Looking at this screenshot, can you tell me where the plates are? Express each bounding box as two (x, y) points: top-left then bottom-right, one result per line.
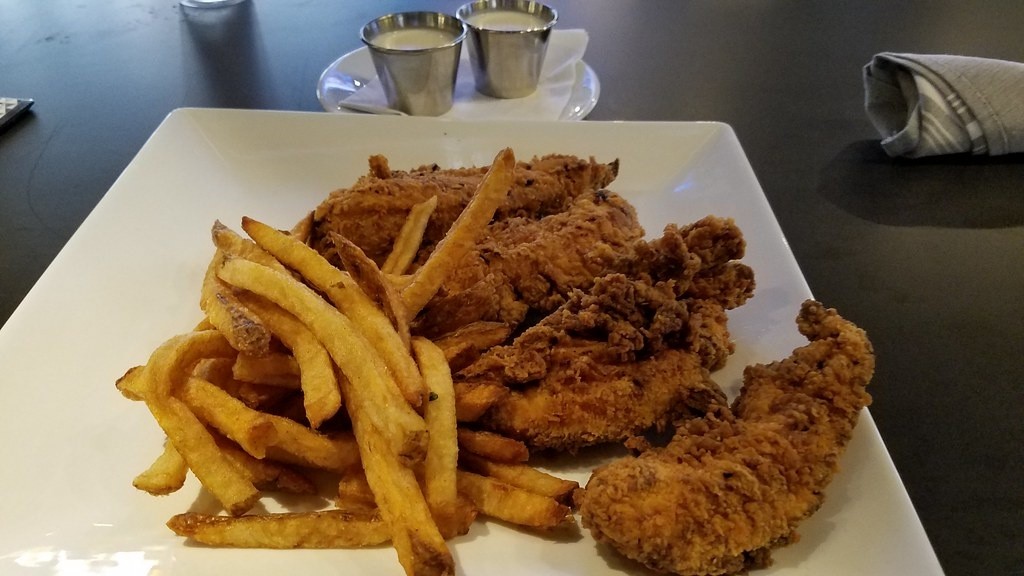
(316, 45), (601, 121)
(0, 108), (946, 576)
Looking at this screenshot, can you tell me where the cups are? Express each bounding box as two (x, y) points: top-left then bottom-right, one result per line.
(456, 0), (559, 99)
(360, 11), (468, 117)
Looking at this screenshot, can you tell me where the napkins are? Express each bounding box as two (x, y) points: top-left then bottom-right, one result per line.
(861, 52), (1024, 158)
(338, 27), (590, 121)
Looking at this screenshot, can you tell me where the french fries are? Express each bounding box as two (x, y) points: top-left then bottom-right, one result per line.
(115, 147), (582, 576)
